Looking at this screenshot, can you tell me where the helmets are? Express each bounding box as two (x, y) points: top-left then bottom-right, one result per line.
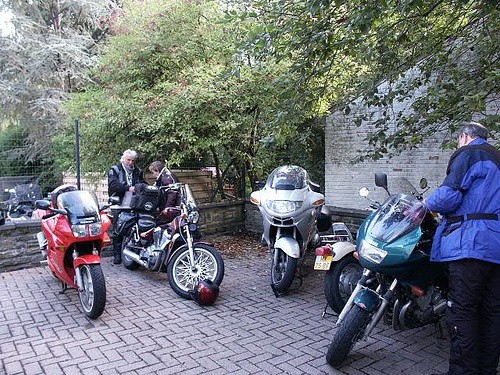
(194, 278), (219, 306)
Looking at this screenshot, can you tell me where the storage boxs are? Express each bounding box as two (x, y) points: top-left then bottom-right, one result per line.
(120, 190), (160, 215)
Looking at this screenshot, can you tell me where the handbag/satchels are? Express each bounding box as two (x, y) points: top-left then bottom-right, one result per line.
(115, 212), (137, 236)
(130, 183), (159, 213)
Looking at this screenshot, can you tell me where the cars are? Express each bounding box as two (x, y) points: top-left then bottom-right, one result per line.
(201, 167), (222, 179)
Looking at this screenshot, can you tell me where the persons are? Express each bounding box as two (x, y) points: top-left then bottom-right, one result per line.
(149, 160), (182, 219)
(419, 123), (500, 375)
(107, 149), (143, 265)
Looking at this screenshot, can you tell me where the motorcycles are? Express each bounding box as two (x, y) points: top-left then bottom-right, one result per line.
(325, 172), (452, 368)
(110, 182), (225, 301)
(313, 177), (431, 318)
(249, 165), (332, 294)
(0, 184), (54, 225)
(34, 184), (121, 320)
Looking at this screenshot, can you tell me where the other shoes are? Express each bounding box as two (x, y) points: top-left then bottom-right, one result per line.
(114, 255), (122, 264)
